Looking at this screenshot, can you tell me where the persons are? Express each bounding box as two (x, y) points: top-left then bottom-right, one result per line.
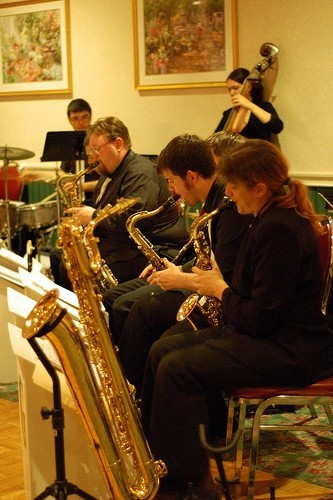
(81, 133), (114, 209)
(116, 133), (254, 396)
(58, 99), (101, 201)
(101, 129), (250, 344)
(214, 68), (284, 142)
(49, 117), (194, 300)
(139, 139), (333, 500)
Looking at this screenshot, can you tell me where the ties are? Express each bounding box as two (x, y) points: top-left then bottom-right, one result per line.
(94, 177), (111, 206)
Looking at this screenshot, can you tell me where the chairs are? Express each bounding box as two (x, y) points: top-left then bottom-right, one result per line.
(225, 215), (333, 499)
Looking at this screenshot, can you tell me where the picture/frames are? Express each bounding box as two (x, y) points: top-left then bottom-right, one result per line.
(131, 0), (241, 91)
(0, 0), (73, 96)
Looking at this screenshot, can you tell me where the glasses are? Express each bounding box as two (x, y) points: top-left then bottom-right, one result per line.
(89, 137), (115, 158)
(69, 116), (90, 122)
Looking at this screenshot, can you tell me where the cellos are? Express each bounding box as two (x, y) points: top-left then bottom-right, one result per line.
(222, 41), (279, 136)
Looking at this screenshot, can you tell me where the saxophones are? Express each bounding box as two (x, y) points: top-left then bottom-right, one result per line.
(19, 192), (169, 499)
(58, 162), (99, 291)
(81, 197), (141, 295)
(175, 194), (236, 335)
(126, 191), (181, 291)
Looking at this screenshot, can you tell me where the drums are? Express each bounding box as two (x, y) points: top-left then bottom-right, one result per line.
(0, 198), (62, 246)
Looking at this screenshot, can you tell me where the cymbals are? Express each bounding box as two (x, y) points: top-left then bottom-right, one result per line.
(0, 145), (36, 163)
